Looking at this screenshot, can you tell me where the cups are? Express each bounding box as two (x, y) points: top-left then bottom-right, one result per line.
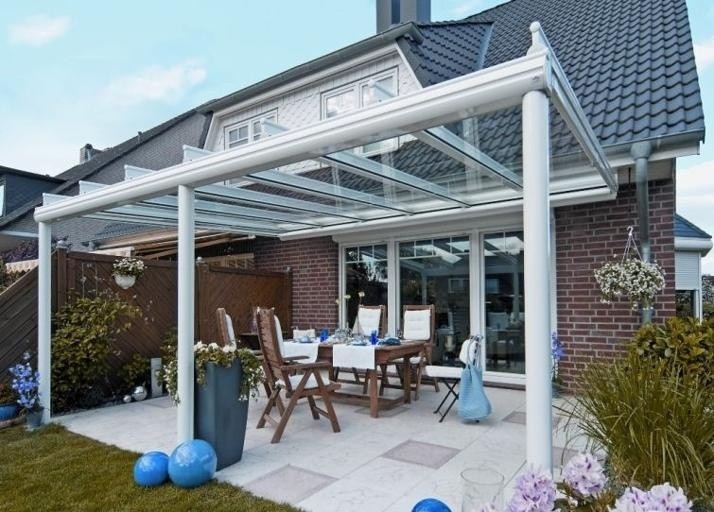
(457, 467), (505, 511)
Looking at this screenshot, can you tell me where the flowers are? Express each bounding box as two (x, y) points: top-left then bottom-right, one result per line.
(152, 341), (267, 403)
(8, 352), (41, 418)
(110, 258), (148, 279)
(594, 255), (667, 312)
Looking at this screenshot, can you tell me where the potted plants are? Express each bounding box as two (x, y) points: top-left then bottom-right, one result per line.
(0, 376), (19, 421)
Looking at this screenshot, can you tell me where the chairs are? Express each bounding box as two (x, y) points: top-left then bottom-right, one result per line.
(425, 337), (479, 422)
(379, 304), (439, 401)
(215, 307), (276, 407)
(256, 306), (341, 443)
(328, 304), (389, 394)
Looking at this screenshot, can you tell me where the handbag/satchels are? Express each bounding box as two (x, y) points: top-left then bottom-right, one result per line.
(458, 367), (490, 420)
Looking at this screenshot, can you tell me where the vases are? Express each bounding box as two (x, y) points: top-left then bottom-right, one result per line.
(114, 274), (136, 290)
(26, 405), (44, 426)
(193, 356), (250, 472)
